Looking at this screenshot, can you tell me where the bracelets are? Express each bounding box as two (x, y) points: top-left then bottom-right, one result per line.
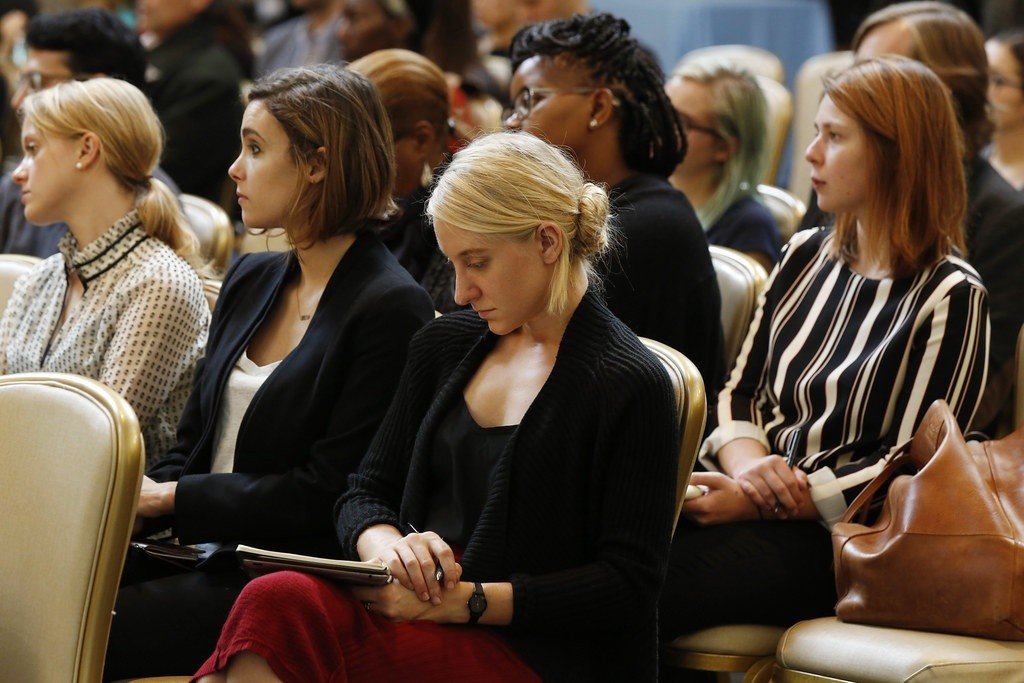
(758, 504), (764, 520)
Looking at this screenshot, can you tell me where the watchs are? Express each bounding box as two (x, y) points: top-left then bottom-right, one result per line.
(467, 582), (487, 625)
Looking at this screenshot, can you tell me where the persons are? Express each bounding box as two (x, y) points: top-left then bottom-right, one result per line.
(0, 0), (1024, 683)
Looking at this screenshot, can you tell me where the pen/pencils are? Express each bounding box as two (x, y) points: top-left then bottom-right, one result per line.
(774, 427), (803, 513)
(406, 520), (444, 581)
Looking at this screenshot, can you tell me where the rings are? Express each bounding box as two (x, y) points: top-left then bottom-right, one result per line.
(364, 602), (371, 611)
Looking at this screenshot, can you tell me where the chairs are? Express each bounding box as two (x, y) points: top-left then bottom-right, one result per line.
(0, 40), (1024, 683)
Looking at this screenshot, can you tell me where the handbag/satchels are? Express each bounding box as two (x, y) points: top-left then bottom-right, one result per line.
(831, 398), (1024, 641)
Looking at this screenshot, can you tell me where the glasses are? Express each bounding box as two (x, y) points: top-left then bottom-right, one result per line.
(677, 107), (727, 141)
(13, 66), (96, 92)
(495, 84), (629, 124)
(988, 69), (1023, 90)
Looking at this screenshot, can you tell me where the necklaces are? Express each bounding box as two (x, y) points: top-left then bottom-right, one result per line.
(297, 283), (321, 321)
